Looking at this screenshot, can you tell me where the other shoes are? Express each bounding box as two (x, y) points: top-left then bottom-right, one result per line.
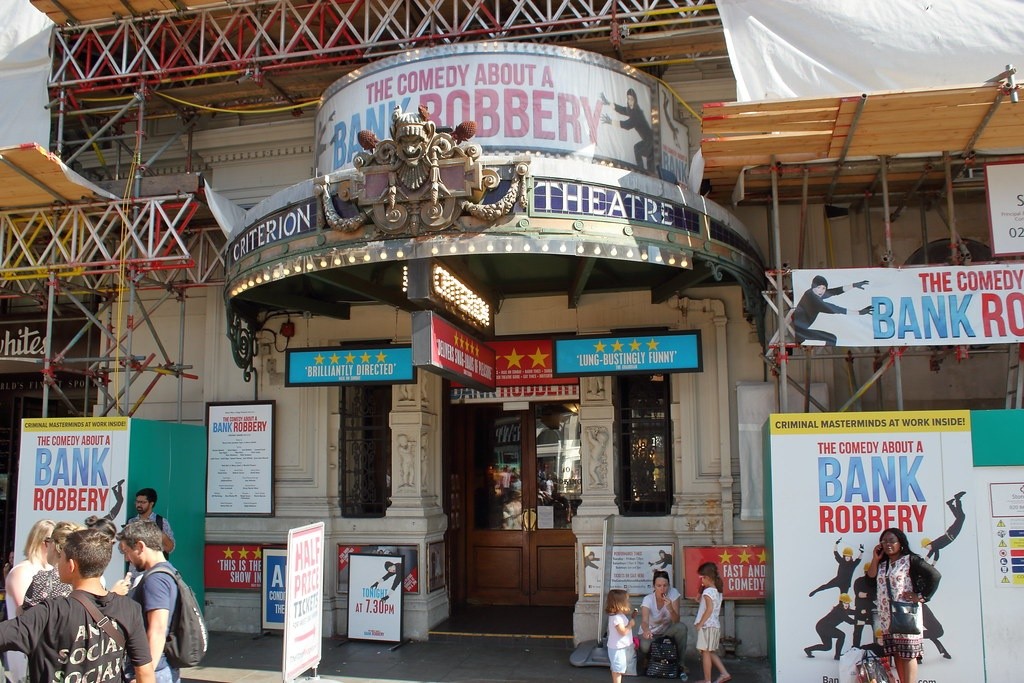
(713, 674), (732, 683)
(804, 648), (815, 657)
(809, 591), (816, 596)
(943, 651), (951, 658)
(955, 492), (966, 499)
(947, 498), (955, 505)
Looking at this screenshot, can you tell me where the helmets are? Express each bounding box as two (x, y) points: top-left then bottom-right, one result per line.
(921, 538), (932, 546)
(864, 562), (872, 571)
(844, 548), (853, 556)
(839, 592), (852, 603)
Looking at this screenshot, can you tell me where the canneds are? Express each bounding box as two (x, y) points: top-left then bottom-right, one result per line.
(680, 672), (687, 682)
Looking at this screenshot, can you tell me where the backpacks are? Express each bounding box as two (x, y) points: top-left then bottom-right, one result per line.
(141, 566), (209, 667)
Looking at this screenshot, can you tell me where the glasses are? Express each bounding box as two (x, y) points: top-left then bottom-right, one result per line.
(699, 575), (706, 579)
(45, 538), (51, 547)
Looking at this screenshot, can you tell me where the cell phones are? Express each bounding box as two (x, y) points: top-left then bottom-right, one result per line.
(124, 572), (132, 582)
(878, 548), (883, 553)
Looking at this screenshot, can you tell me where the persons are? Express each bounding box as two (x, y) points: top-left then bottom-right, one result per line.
(102, 479), (126, 520)
(693, 560), (732, 682)
(604, 588), (634, 683)
(488, 462), (559, 496)
(125, 488), (177, 589)
(790, 273), (869, 347)
(636, 570), (690, 673)
(802, 490), (968, 664)
(0, 514), (183, 682)
(660, 87), (680, 148)
(599, 88), (656, 175)
(862, 527), (941, 683)
(368, 559), (402, 602)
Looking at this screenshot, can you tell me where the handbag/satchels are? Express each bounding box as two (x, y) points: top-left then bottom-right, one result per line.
(889, 599), (921, 635)
(838, 647), (898, 683)
(646, 636), (679, 678)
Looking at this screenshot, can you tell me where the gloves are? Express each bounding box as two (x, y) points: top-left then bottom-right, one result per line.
(601, 113), (612, 124)
(370, 582), (379, 589)
(648, 562), (654, 566)
(328, 110), (336, 122)
(858, 305), (875, 316)
(865, 619), (875, 626)
(653, 568), (657, 572)
(853, 280), (869, 290)
(859, 544), (864, 553)
(599, 91), (610, 106)
(835, 537), (842, 545)
(381, 595), (389, 602)
(330, 135), (337, 146)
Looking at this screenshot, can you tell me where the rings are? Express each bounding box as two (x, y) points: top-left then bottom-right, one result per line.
(647, 634), (650, 637)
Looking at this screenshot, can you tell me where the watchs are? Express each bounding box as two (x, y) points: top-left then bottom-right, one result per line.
(917, 593), (927, 604)
